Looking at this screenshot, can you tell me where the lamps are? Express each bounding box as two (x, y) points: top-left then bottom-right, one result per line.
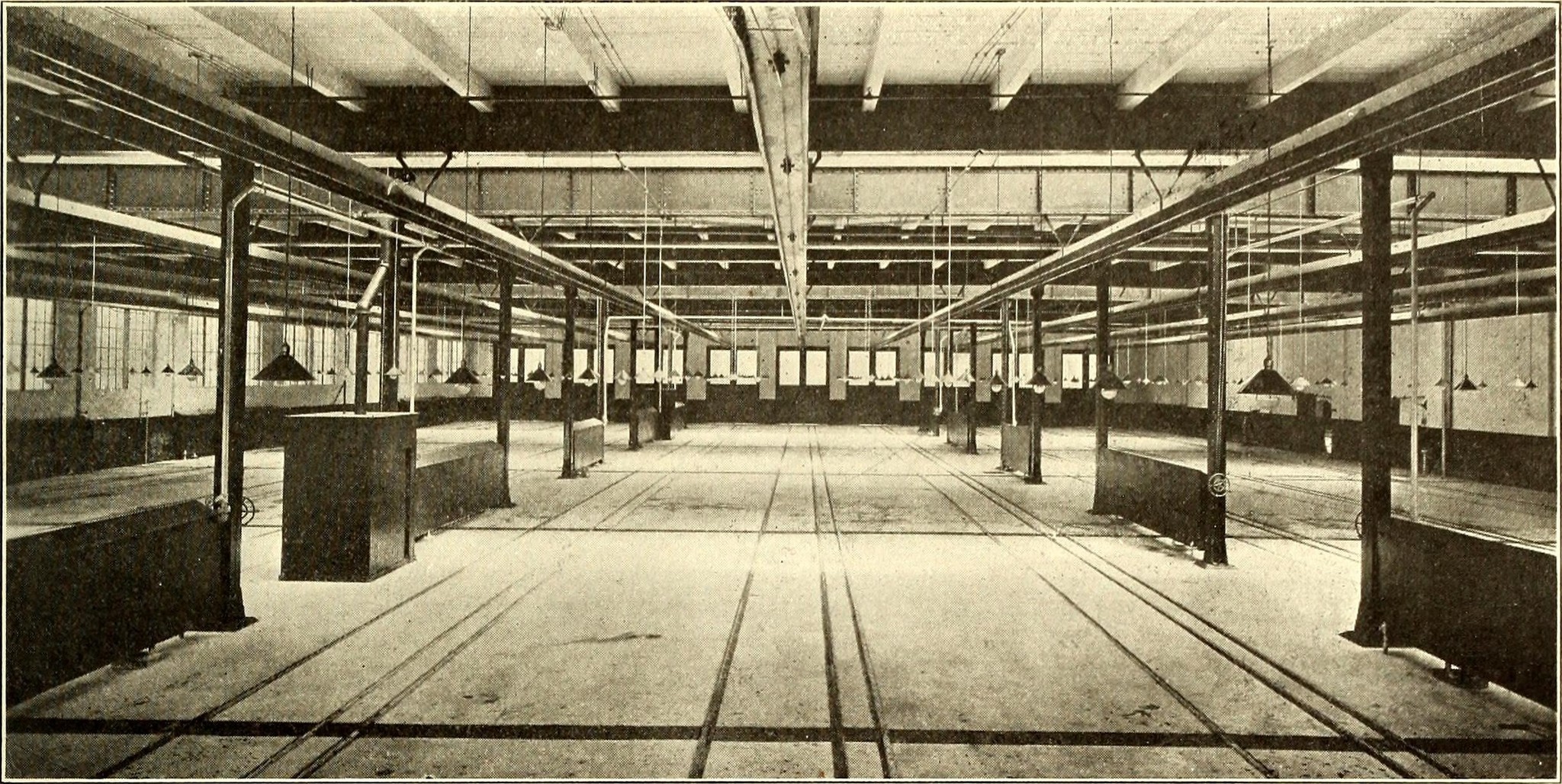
(7, 7), (1556, 398)
